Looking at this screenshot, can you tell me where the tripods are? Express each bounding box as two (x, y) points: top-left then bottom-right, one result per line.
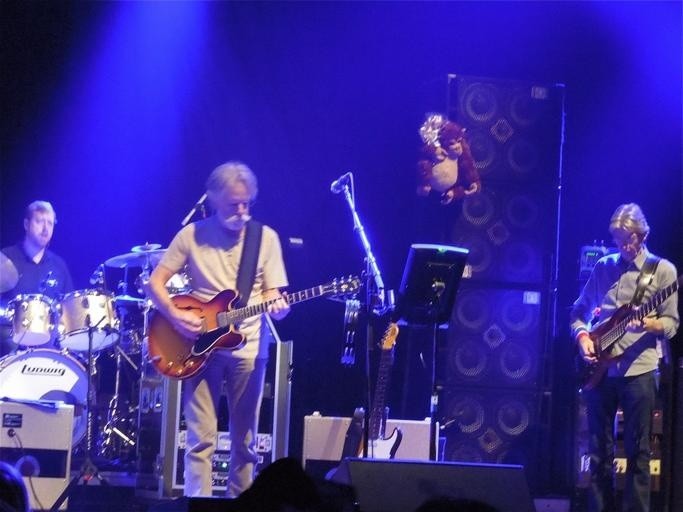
(73, 255), (149, 458)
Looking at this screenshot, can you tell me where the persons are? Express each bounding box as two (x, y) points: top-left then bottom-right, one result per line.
(0, 201), (75, 358)
(569, 202), (680, 511)
(144, 162), (290, 500)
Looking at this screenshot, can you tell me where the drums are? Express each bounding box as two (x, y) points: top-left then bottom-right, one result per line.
(7, 294), (57, 346)
(55, 287), (121, 353)
(0, 348), (96, 451)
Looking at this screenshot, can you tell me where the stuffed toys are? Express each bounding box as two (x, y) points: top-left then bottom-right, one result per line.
(417, 114), (481, 205)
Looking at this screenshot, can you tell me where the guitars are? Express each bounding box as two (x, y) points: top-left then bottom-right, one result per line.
(574, 273), (683, 394)
(325, 324), (402, 486)
(149, 274), (364, 380)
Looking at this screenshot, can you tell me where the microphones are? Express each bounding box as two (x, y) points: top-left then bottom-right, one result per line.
(181, 190), (208, 226)
(39, 272), (51, 293)
(331, 173), (350, 194)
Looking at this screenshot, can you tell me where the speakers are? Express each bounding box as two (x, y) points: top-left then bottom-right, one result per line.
(436, 72), (566, 500)
(328, 457), (536, 512)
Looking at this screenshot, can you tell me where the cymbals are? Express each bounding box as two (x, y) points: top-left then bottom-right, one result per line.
(131, 243), (161, 252)
(104, 249), (167, 268)
